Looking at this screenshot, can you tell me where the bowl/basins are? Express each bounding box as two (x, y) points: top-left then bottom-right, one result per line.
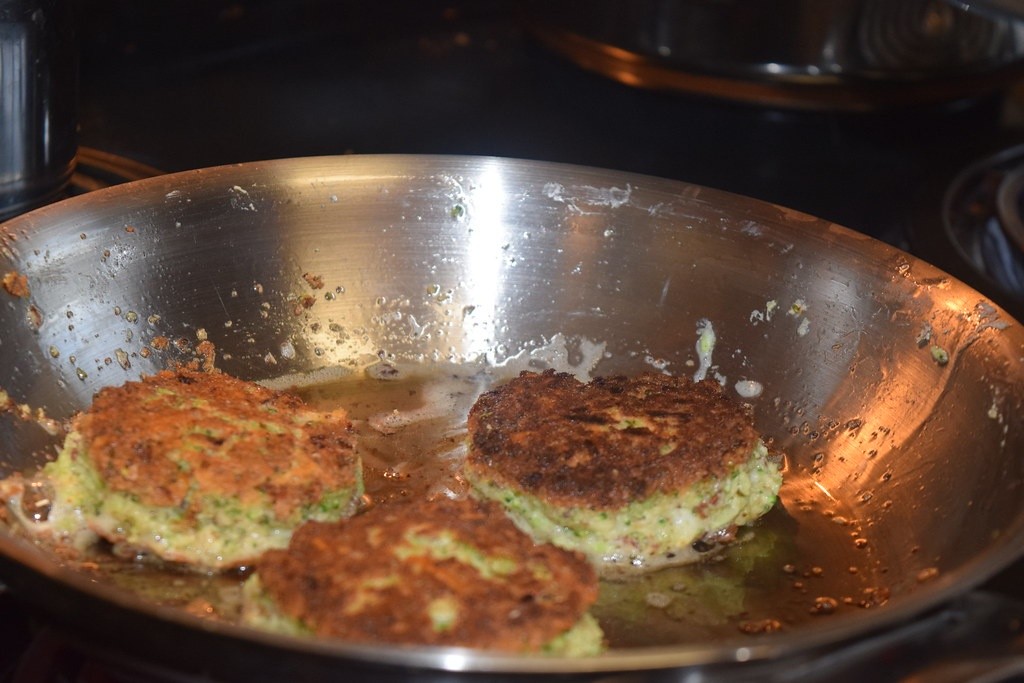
(0, 154), (1024, 683)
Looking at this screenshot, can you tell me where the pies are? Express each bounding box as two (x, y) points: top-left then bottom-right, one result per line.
(46, 367), (364, 575)
(463, 370), (784, 569)
(243, 494), (609, 657)
